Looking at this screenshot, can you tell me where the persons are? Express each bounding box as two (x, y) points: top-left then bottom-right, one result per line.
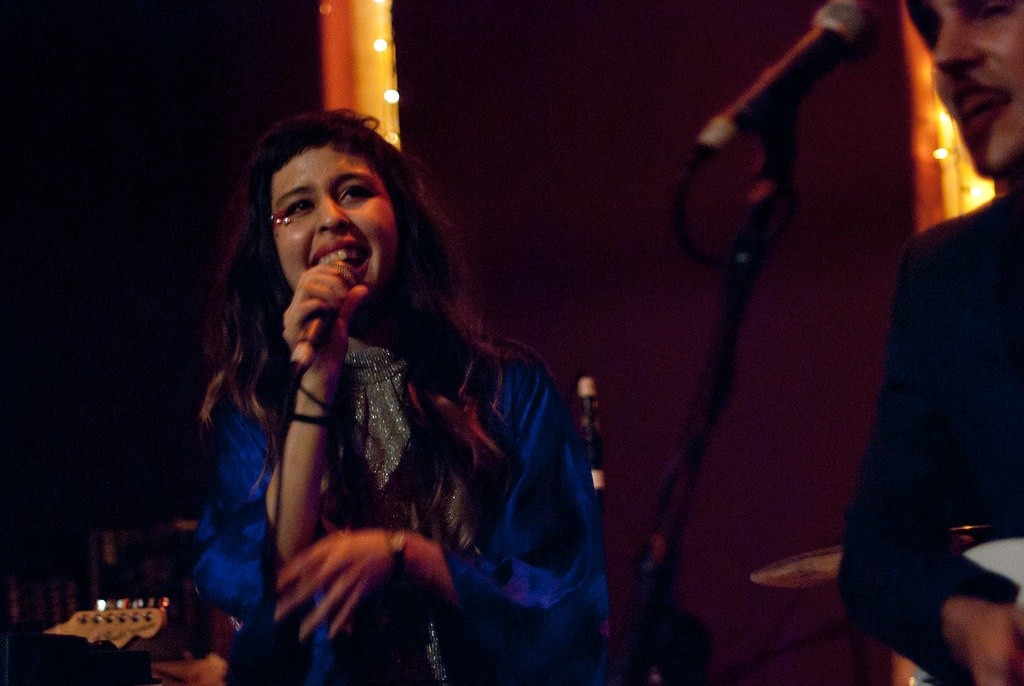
(836, 0), (1024, 686)
(193, 108), (610, 686)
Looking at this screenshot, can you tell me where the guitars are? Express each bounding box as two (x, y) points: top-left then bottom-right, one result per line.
(42, 595), (171, 649)
(892, 537), (1024, 686)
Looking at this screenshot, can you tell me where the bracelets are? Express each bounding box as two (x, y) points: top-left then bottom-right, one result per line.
(389, 527), (406, 581)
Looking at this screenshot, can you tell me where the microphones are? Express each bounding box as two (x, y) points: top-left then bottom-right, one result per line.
(287, 260), (358, 379)
(579, 373), (607, 513)
(691, 0), (881, 164)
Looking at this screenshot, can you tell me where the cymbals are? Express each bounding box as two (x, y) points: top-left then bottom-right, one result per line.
(749, 523), (994, 588)
